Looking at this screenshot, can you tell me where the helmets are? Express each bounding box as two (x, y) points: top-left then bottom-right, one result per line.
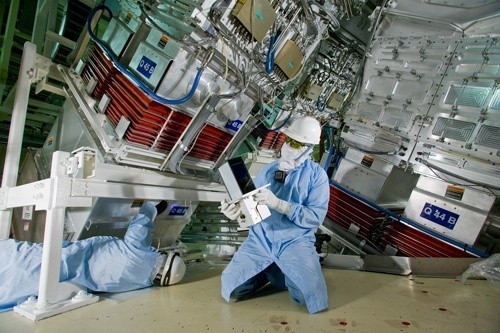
(160, 250), (186, 285)
(284, 116), (321, 144)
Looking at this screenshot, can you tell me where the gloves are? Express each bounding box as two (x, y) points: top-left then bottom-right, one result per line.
(221, 198), (242, 220)
(253, 188), (288, 215)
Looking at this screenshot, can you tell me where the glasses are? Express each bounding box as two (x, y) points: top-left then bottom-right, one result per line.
(286, 136), (311, 149)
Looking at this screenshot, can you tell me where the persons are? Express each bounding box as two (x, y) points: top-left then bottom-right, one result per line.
(0, 199), (186, 312)
(221, 116), (330, 313)
